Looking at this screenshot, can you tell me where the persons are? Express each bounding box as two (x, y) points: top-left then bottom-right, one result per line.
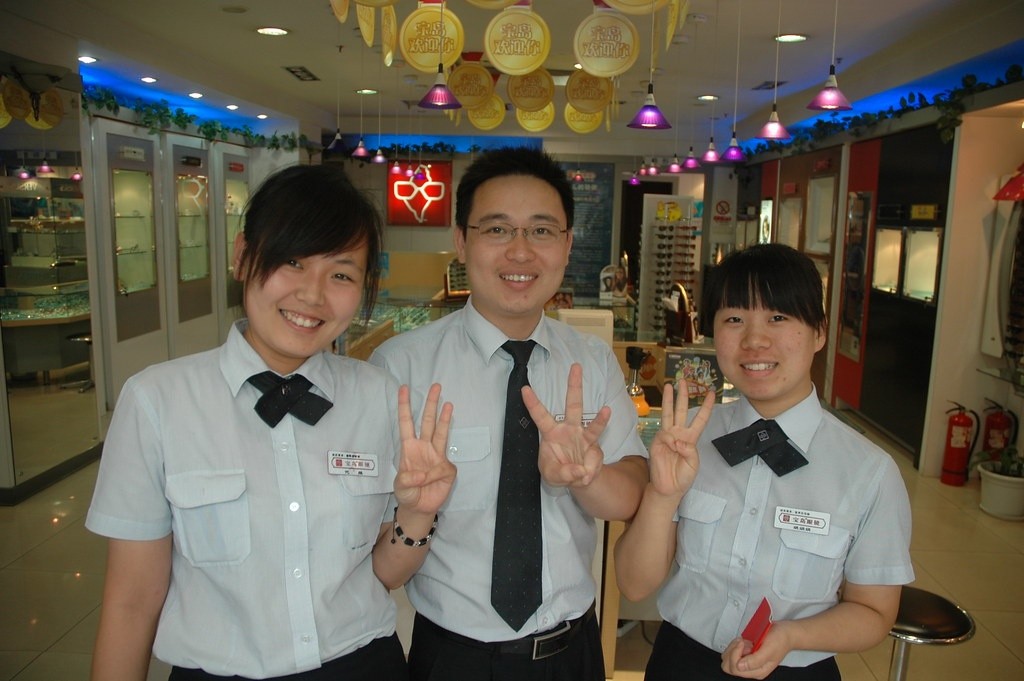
(367, 143), (653, 681)
(613, 243), (916, 681)
(84, 164), (457, 681)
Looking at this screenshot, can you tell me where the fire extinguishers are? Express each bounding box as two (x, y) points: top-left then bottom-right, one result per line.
(941, 400), (981, 487)
(978, 396), (1019, 482)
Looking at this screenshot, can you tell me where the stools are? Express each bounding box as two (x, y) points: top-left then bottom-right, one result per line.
(890, 585), (976, 681)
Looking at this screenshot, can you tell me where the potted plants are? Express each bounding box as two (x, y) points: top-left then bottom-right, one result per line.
(968, 443), (1024, 522)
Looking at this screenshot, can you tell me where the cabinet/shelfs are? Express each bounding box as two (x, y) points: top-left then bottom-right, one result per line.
(0, 176), (92, 376)
(601, 343), (726, 680)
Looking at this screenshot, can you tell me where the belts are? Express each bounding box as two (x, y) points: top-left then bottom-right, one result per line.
(415, 598), (596, 660)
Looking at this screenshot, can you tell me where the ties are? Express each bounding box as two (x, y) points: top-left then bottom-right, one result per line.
(491, 339), (543, 634)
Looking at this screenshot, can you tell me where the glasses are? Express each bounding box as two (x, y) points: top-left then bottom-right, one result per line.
(0, 209), (246, 320)
(464, 222), (568, 245)
(360, 257), (470, 326)
(633, 218), (697, 330)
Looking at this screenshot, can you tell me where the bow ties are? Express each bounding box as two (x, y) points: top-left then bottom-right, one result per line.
(710, 419), (809, 477)
(247, 371), (334, 429)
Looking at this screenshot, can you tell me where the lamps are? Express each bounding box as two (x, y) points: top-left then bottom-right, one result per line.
(328, 0), (462, 180)
(628, 0), (851, 184)
(16, 118), (83, 182)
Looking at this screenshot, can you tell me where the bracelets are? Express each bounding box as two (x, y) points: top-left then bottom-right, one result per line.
(391, 506), (438, 547)
